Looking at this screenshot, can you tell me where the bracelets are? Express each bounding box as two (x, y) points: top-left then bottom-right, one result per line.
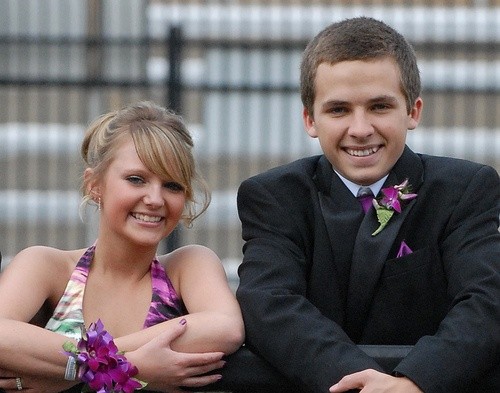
(63, 333), (83, 382)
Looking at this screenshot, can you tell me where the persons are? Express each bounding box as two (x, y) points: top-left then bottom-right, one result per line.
(0, 101), (245, 393)
(237, 16), (499, 393)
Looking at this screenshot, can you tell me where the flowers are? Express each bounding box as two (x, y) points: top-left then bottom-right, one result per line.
(60, 316), (148, 393)
(371, 178), (418, 236)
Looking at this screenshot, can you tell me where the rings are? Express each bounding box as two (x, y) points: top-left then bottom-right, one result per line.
(16, 377), (22, 390)
